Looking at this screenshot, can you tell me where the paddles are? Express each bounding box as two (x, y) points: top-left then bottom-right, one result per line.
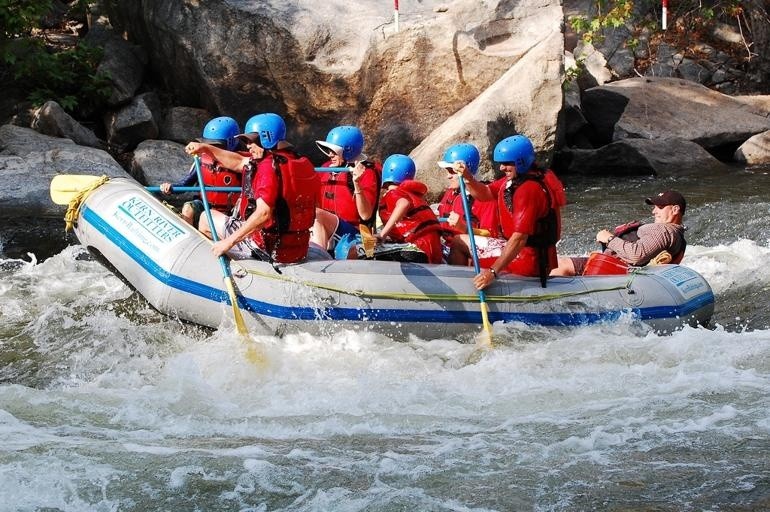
(189, 145), (265, 368)
(456, 163), (499, 351)
(50, 174), (242, 205)
(359, 224), (392, 259)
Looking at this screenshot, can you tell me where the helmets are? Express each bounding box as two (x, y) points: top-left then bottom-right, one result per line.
(435, 142), (481, 180)
(200, 114), (242, 153)
(492, 134), (537, 175)
(316, 124), (366, 161)
(380, 152), (417, 190)
(233, 110), (288, 150)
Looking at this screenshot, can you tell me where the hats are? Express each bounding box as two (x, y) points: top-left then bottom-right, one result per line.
(643, 189), (687, 210)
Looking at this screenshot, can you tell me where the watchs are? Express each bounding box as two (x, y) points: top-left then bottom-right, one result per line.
(607, 235), (615, 246)
(489, 267), (497, 278)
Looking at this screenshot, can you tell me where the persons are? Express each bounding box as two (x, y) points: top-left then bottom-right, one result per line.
(186, 113), (321, 264)
(454, 134), (565, 291)
(436, 143), (497, 249)
(160, 116), (246, 229)
(550, 191), (688, 276)
(312, 125), (383, 260)
(375, 153), (443, 267)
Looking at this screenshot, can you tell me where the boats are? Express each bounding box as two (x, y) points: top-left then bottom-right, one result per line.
(70, 177), (715, 336)
